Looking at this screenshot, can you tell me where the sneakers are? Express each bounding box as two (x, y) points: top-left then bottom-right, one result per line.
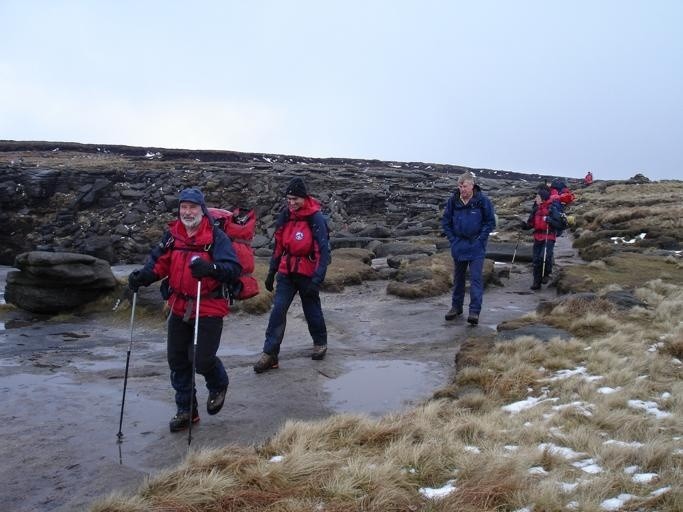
(254, 352), (279, 371)
(311, 341), (328, 358)
(168, 405), (201, 431)
(443, 302), (465, 320)
(206, 385), (228, 414)
(467, 310), (480, 326)
(530, 275), (553, 290)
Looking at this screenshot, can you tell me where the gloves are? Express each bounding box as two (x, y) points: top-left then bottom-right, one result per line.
(544, 216), (551, 223)
(189, 258), (216, 278)
(127, 268), (149, 292)
(304, 276), (321, 297)
(521, 221), (529, 231)
(265, 273), (276, 292)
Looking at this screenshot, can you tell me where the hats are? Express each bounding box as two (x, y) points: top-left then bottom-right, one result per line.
(179, 190), (203, 206)
(286, 178), (309, 200)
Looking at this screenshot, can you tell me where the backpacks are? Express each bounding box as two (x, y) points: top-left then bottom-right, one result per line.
(216, 209), (259, 299)
(552, 187), (575, 232)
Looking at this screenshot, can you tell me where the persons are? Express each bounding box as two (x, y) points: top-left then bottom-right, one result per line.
(254, 177), (329, 373)
(442, 172), (496, 324)
(520, 178), (567, 290)
(585, 171), (592, 185)
(129, 189), (240, 431)
